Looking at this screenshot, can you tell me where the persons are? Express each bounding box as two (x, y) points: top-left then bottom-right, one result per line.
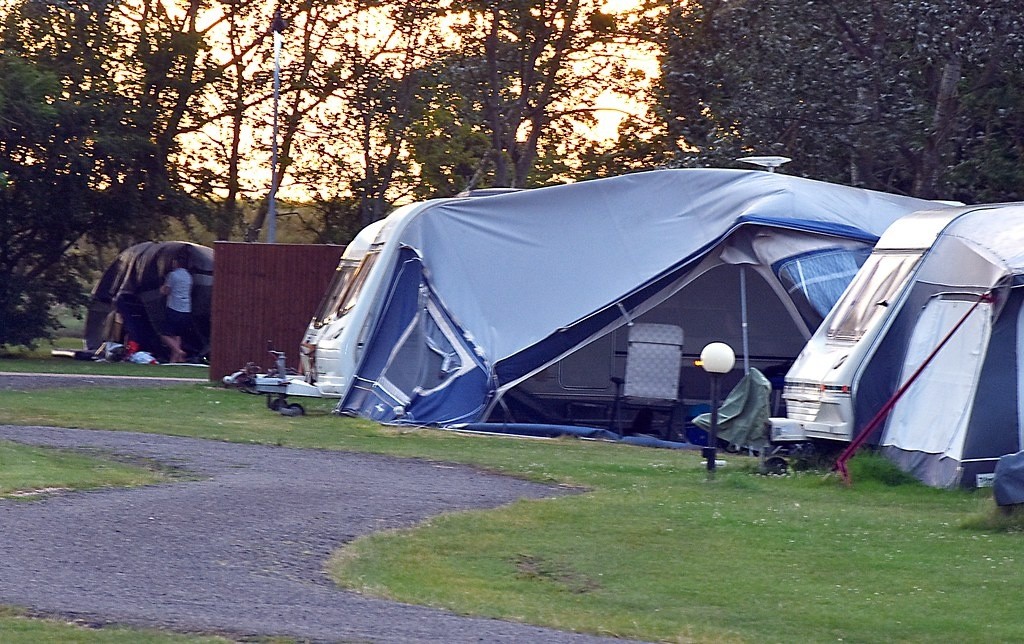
(156, 255), (192, 363)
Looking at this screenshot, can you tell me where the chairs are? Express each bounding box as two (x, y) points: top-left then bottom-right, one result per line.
(610, 324), (685, 441)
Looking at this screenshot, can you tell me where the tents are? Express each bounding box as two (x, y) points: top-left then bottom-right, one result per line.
(338, 168), (967, 452)
(85, 239), (215, 365)
